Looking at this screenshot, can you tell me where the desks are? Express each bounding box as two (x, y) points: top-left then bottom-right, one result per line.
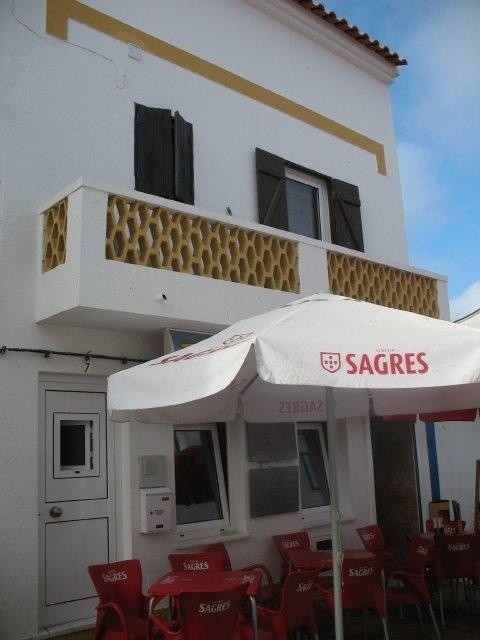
(153, 570), (263, 597)
(284, 550), (383, 573)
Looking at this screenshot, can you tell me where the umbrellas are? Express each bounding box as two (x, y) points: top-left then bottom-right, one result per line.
(104, 292), (479, 640)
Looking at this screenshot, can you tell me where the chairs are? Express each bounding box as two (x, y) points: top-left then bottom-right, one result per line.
(269, 533), (311, 552)
(348, 525), (394, 550)
(174, 550), (219, 569)
(260, 572), (328, 635)
(83, 561), (158, 636)
(437, 537), (478, 615)
(153, 585), (251, 638)
(323, 557), (392, 639)
(389, 550), (447, 637)
